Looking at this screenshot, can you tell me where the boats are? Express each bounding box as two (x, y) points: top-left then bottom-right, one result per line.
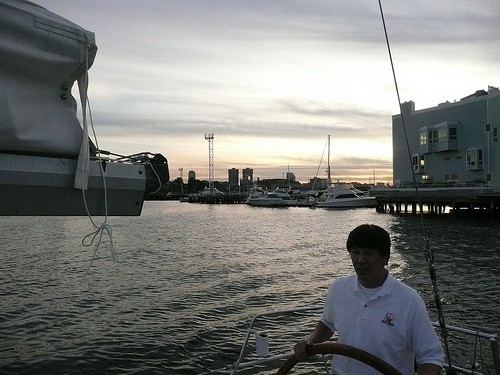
(181, 180), (377, 210)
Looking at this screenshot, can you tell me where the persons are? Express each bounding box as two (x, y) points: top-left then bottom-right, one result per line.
(294, 224), (444, 375)
